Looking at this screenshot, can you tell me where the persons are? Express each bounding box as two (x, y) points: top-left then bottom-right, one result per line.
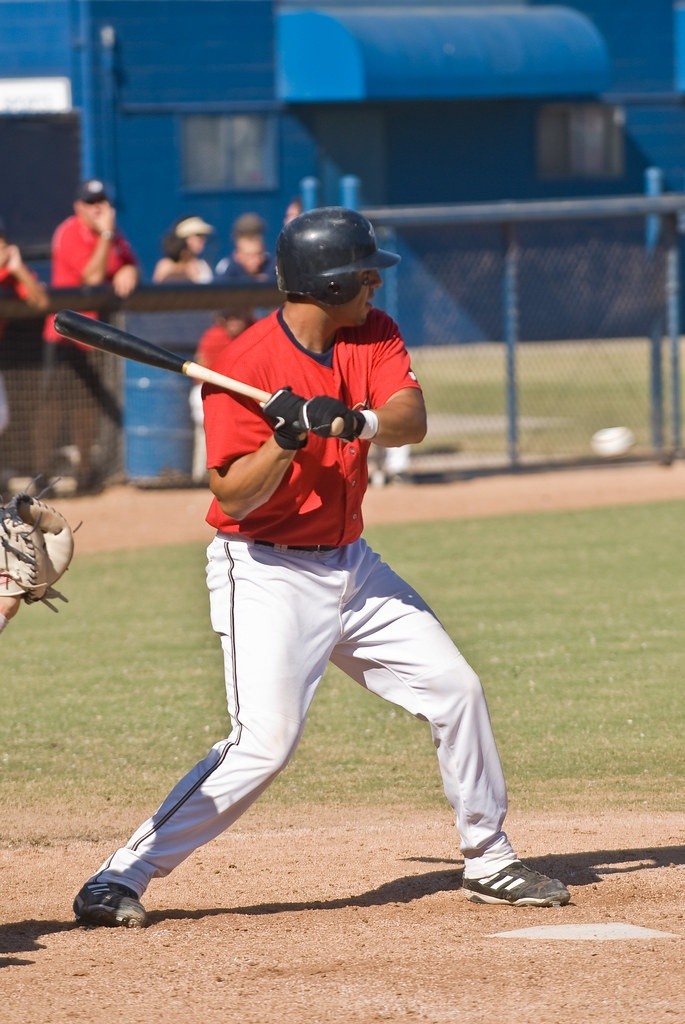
(0, 177), (301, 493)
(73, 208), (571, 928)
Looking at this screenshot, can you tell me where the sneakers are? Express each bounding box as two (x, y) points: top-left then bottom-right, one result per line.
(72, 881), (145, 929)
(462, 859), (570, 907)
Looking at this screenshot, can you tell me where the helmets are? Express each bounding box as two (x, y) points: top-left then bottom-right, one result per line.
(276, 206), (401, 306)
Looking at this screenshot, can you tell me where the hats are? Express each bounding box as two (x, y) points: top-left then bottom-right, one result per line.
(175, 216), (214, 237)
(76, 181), (106, 200)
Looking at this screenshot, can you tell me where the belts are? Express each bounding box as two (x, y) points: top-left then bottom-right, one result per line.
(254, 540), (338, 552)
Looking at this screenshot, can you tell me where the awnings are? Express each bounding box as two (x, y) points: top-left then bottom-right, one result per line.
(275, 7), (609, 100)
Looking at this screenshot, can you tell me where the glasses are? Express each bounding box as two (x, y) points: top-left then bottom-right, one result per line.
(85, 198), (104, 204)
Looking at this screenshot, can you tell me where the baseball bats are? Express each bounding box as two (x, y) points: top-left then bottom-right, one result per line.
(54, 306), (348, 439)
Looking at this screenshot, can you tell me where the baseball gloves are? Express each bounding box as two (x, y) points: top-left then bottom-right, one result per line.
(0, 492), (77, 615)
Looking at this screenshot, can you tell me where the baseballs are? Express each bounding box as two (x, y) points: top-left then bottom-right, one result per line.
(591, 426), (639, 462)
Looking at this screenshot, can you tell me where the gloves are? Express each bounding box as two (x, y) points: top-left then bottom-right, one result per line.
(298, 395), (378, 442)
(262, 386), (308, 450)
